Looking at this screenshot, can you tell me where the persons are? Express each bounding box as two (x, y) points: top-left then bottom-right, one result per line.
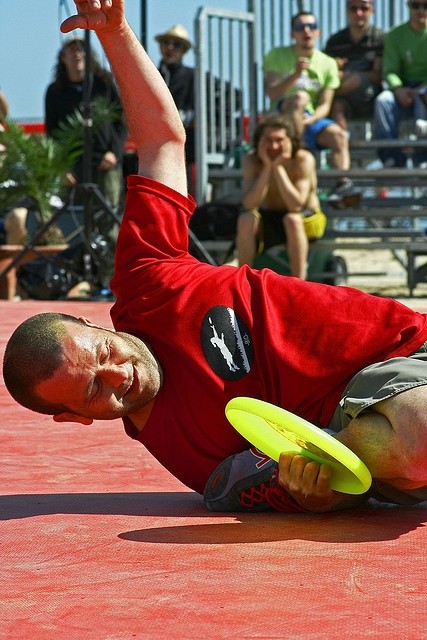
(154, 25), (243, 194)
(238, 118), (326, 280)
(372, 0), (427, 169)
(0, 94), (35, 303)
(323, 0), (387, 139)
(45, 38), (128, 299)
(3, 0), (426, 516)
(263, 12), (364, 209)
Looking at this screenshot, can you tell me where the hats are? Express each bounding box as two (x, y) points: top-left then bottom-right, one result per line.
(155, 24), (191, 48)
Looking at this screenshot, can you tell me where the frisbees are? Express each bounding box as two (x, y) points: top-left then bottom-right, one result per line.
(224, 395), (372, 497)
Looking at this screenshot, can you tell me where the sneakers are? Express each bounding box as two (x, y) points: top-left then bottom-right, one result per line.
(203, 429), (336, 514)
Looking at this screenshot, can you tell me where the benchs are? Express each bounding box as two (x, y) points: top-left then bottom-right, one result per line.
(0, 246), (68, 300)
(199, 80), (427, 294)
